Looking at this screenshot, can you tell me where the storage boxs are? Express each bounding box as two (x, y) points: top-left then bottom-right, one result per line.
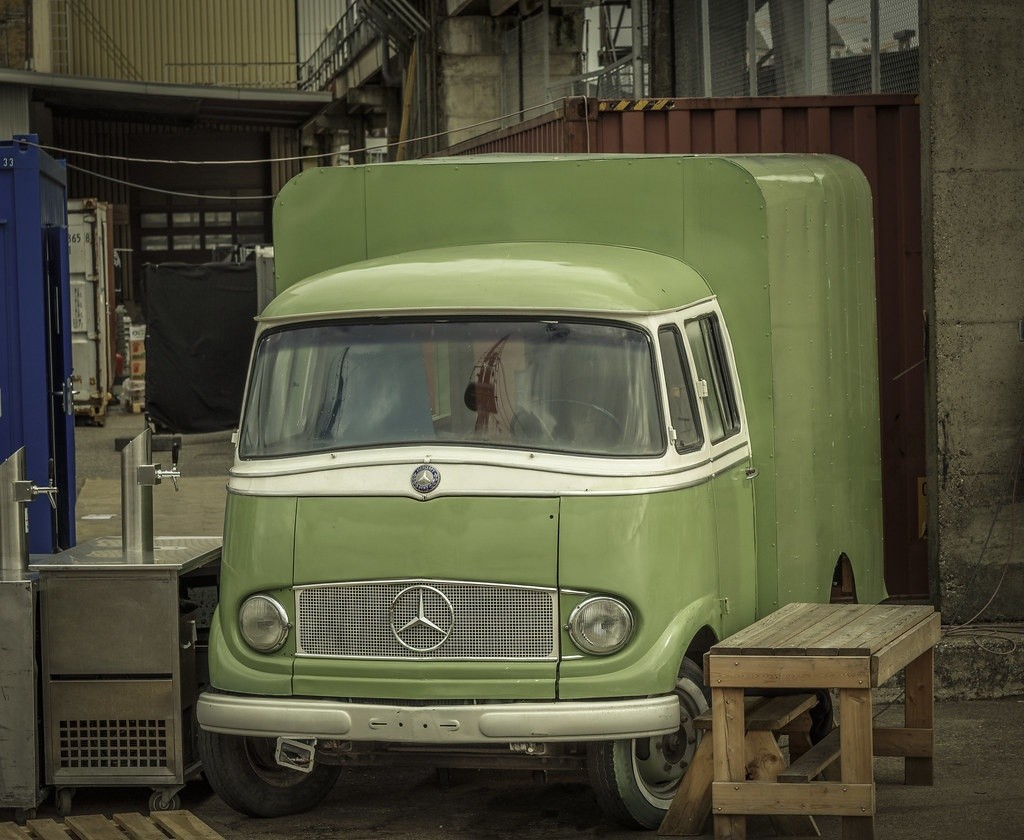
(432, 94), (927, 601)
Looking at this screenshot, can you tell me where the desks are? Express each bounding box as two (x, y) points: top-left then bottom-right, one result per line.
(703, 601), (941, 840)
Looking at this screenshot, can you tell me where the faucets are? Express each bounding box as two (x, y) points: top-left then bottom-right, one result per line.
(30, 457), (60, 510)
(154, 442), (181, 492)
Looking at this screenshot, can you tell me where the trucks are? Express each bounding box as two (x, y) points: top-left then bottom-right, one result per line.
(196, 152), (886, 830)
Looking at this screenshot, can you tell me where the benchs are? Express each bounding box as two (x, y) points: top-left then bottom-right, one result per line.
(654, 694), (822, 837)
